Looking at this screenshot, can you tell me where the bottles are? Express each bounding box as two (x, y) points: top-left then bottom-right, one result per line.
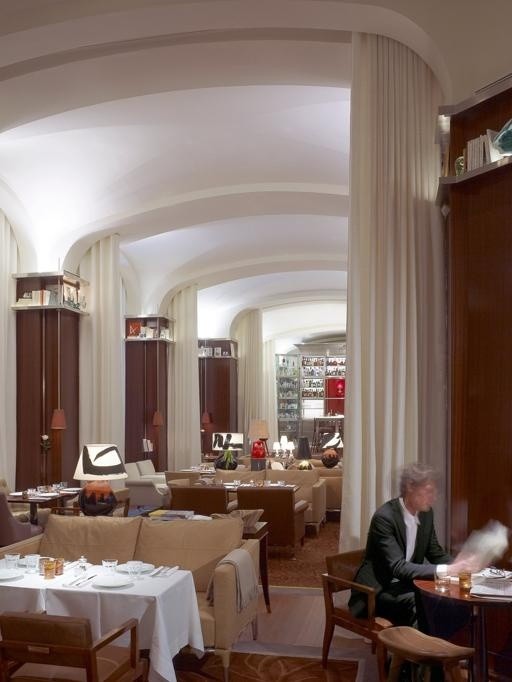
(23, 490), (28, 498)
(279, 380), (323, 388)
(301, 388), (324, 397)
(278, 355), (346, 376)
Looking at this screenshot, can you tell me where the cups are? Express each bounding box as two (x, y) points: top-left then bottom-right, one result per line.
(279, 390), (296, 409)
(4, 553), (144, 581)
(457, 567), (472, 590)
(432, 569), (449, 592)
(215, 479), (286, 486)
(28, 481), (68, 497)
(223, 351), (229, 357)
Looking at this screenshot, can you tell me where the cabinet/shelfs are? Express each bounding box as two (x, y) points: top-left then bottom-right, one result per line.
(10, 271), (92, 317)
(437, 76), (512, 187)
(294, 341), (346, 401)
(269, 352), (301, 442)
(121, 314), (177, 344)
(197, 336), (240, 360)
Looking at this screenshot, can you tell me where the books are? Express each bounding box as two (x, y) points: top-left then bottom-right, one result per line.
(148, 509), (194, 521)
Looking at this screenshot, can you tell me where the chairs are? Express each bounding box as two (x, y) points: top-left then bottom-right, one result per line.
(1, 492), (45, 549)
(164, 468), (328, 560)
(312, 414), (345, 455)
(121, 459), (173, 505)
(1, 513), (267, 681)
(288, 459), (341, 515)
(315, 546), (398, 670)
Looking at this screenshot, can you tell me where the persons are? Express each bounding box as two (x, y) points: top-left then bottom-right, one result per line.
(348, 462), (470, 682)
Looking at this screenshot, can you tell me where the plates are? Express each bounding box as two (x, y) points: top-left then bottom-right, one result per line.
(117, 563), (155, 573)
(93, 575), (133, 588)
(1, 567), (23, 581)
(8, 491), (23, 497)
(60, 487), (82, 494)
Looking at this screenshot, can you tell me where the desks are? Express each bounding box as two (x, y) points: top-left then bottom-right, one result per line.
(7, 485), (83, 526)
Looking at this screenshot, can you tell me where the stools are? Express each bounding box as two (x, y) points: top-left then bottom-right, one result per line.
(376, 621), (478, 682)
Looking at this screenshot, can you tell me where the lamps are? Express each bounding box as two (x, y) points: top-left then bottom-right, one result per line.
(272, 434), (297, 461)
(210, 430), (245, 472)
(72, 441), (129, 519)
